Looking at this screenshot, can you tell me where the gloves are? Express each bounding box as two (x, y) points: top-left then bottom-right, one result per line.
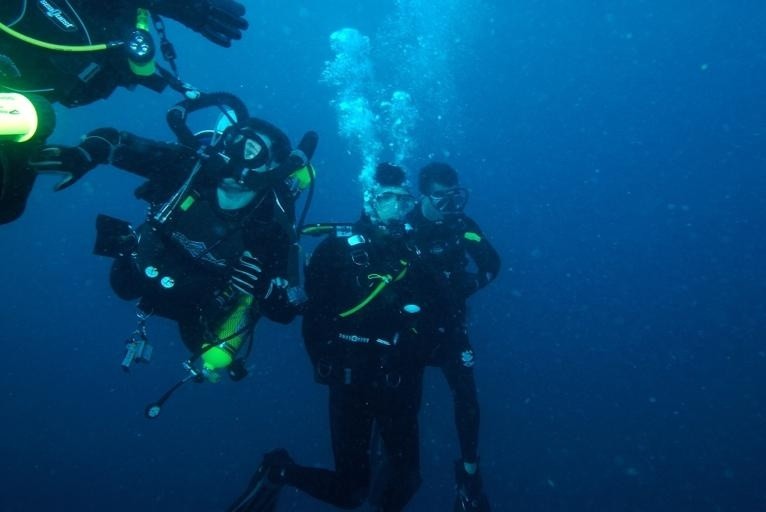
(228, 254), (274, 299)
(27, 141), (93, 192)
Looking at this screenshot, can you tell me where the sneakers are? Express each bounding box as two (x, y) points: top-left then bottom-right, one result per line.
(260, 446), (289, 501)
(454, 461), (482, 511)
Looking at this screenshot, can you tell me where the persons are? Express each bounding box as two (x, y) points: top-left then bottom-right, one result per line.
(224, 160), (442, 511)
(396, 163), (503, 512)
(0, 1), (250, 229)
(22, 91), (309, 354)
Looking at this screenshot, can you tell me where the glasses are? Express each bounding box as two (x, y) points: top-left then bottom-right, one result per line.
(375, 191), (418, 217)
(427, 189), (465, 212)
(222, 128), (269, 170)
